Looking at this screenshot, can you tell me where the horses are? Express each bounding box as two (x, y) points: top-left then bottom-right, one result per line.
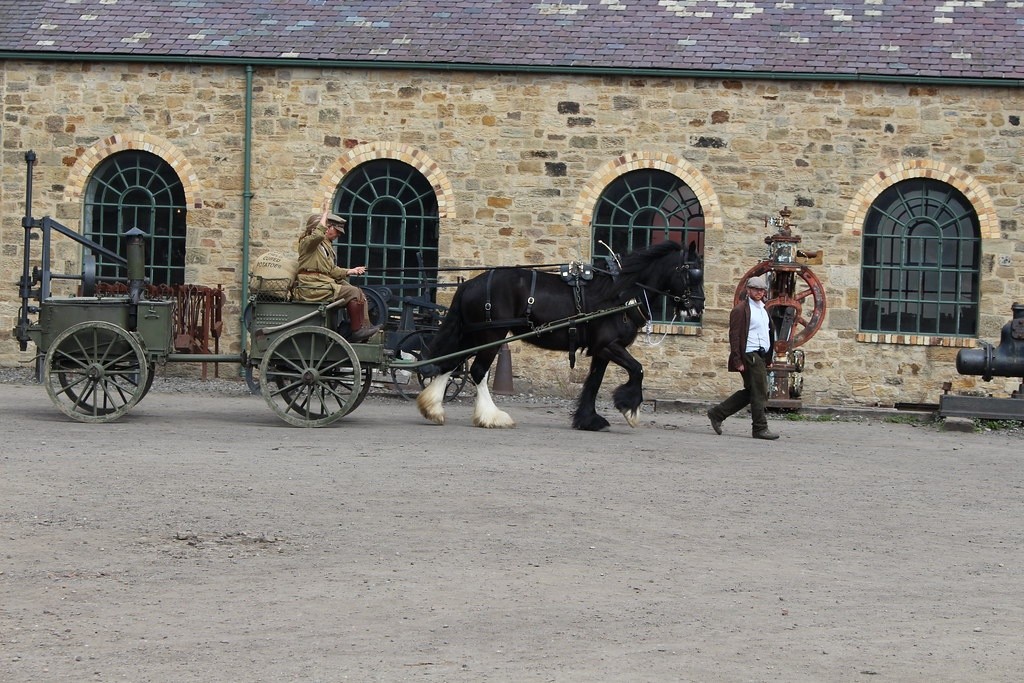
(415, 240), (707, 432)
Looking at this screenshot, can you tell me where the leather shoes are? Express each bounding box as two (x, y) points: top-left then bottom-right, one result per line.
(708, 409), (722, 435)
(752, 428), (779, 440)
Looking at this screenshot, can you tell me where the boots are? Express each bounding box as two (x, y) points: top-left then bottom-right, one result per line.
(348, 301), (384, 342)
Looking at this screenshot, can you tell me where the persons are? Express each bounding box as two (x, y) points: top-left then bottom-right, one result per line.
(323, 214), (368, 342)
(708, 277), (780, 439)
(298, 198), (385, 342)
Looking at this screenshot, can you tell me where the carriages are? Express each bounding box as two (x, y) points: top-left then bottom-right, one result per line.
(11, 150), (706, 434)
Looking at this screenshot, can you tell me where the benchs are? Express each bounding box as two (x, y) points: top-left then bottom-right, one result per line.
(292, 299), (330, 305)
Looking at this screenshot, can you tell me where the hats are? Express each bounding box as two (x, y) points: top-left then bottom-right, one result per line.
(327, 213), (347, 235)
(747, 277), (767, 289)
